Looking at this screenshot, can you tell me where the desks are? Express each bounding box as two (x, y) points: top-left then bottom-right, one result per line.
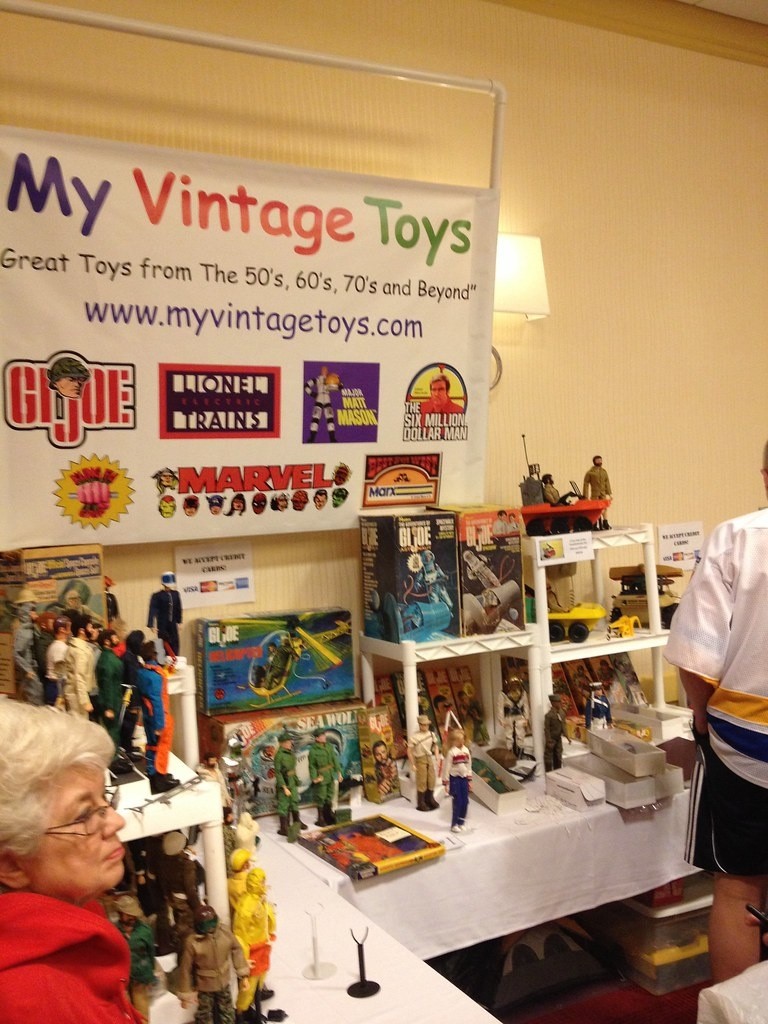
(264, 770), (705, 962)
(108, 662), (232, 926)
(357, 628), (545, 774)
(144, 825), (503, 1024)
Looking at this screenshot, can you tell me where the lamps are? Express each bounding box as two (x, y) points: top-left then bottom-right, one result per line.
(489, 229), (552, 389)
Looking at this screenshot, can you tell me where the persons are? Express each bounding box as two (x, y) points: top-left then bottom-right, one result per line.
(541, 474), (577, 506)
(664, 440), (768, 986)
(583, 456), (615, 532)
(491, 511), (520, 534)
(198, 737), (261, 827)
(499, 676), (574, 774)
(274, 728), (343, 837)
(408, 716), (472, 834)
(586, 682), (612, 735)
(112, 806), (279, 1024)
(743, 908), (768, 947)
(0, 696), (150, 1024)
(12, 571), (183, 795)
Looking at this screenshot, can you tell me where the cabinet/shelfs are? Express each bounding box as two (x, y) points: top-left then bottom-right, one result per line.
(490, 523), (671, 767)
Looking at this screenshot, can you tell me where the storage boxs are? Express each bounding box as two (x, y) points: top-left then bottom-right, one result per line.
(580, 871), (715, 995)
(561, 753), (658, 810)
(194, 699), (365, 816)
(0, 542), (109, 698)
(390, 669), (443, 752)
(466, 741), (527, 814)
(654, 763), (685, 798)
(587, 728), (667, 777)
(430, 503), (526, 639)
(355, 706), (401, 803)
(423, 668), (468, 747)
(545, 766), (607, 811)
(585, 656), (629, 709)
(357, 513), (463, 645)
(567, 712), (652, 747)
(449, 665), (490, 745)
(564, 659), (594, 713)
(610, 652), (648, 704)
(611, 704), (684, 740)
(374, 675), (407, 760)
(552, 663), (579, 715)
(113, 762), (152, 808)
(194, 605), (356, 717)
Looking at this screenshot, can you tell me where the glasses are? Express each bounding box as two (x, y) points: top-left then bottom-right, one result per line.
(47, 785), (120, 835)
